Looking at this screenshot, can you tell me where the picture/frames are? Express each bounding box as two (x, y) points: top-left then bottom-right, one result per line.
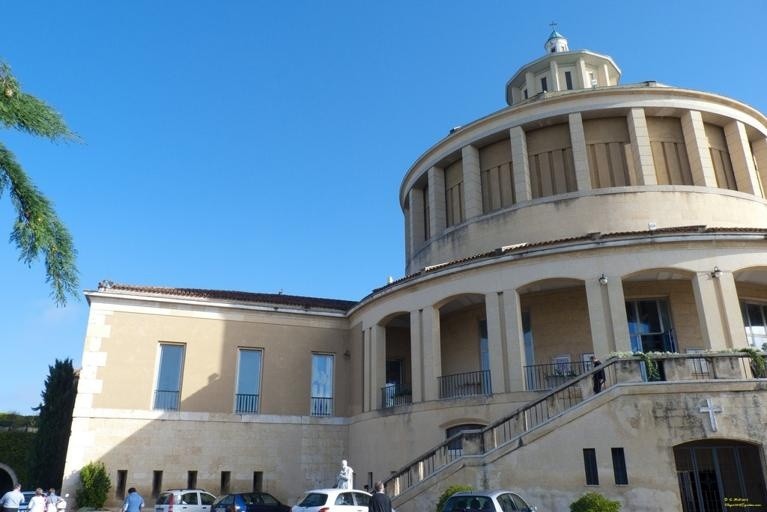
(551, 352), (595, 376)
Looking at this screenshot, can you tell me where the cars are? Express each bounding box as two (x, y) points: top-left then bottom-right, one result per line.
(154, 488), (398, 512)
(436, 487), (538, 512)
(18, 490), (49, 512)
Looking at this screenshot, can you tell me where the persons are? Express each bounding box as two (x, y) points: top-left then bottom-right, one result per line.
(44, 488), (64, 512)
(27, 487), (47, 512)
(385, 374), (397, 408)
(0, 483), (25, 512)
(121, 487), (145, 512)
(367, 481), (392, 512)
(336, 459), (354, 490)
(590, 355), (607, 395)
